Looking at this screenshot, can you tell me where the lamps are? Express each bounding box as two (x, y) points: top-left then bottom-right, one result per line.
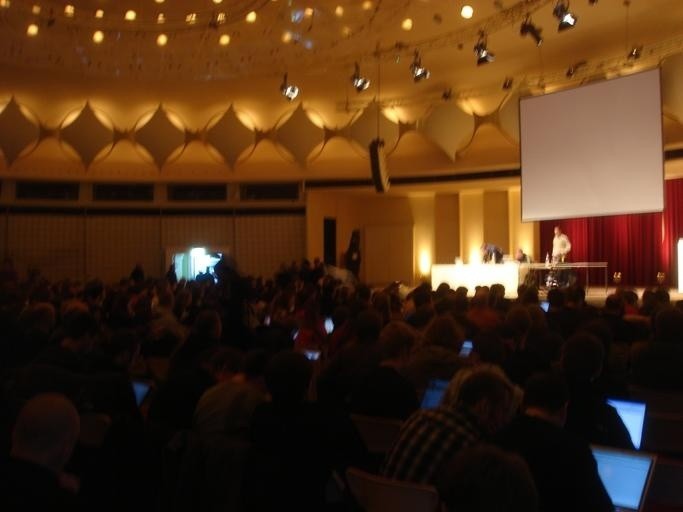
(518, 11), (542, 45)
(553, 1), (578, 31)
(409, 47), (430, 82)
(280, 73), (298, 101)
(474, 35), (494, 66)
(352, 62), (369, 92)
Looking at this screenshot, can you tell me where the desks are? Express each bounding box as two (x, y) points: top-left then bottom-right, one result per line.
(431, 263), (520, 299)
(519, 263), (609, 297)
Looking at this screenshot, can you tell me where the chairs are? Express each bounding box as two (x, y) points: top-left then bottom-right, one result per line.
(449, 445), (540, 511)
(349, 413), (403, 454)
(344, 467), (439, 511)
